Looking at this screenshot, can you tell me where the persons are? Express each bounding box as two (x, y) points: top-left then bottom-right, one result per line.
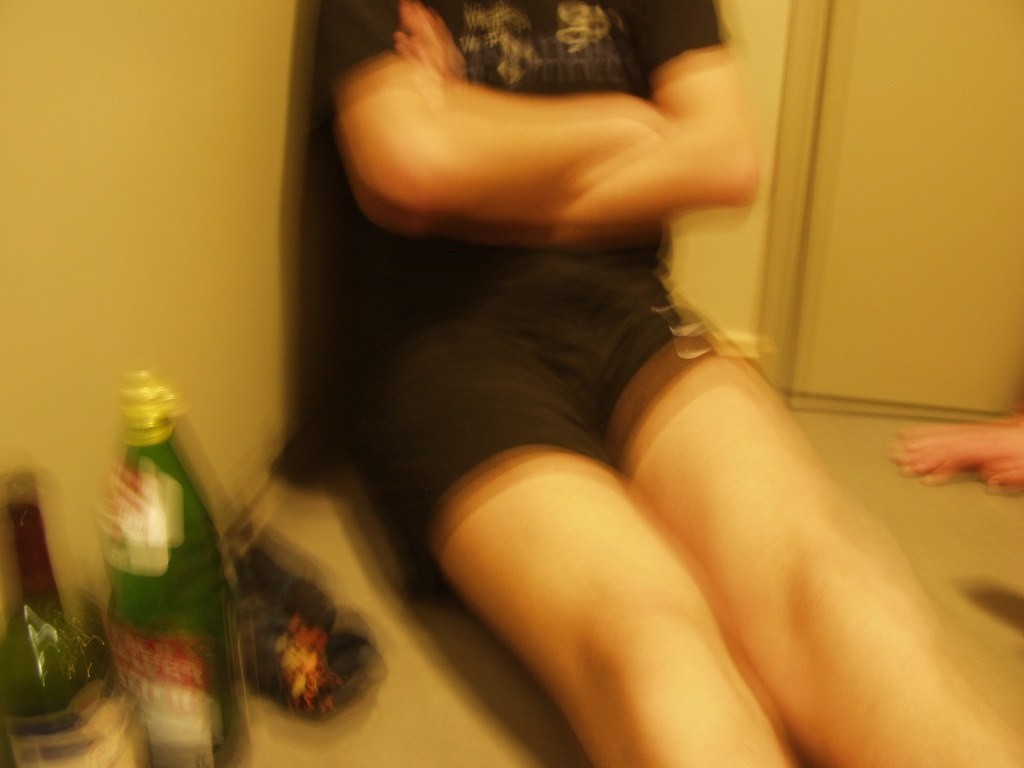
(277, 0), (1016, 767)
(894, 422), (1024, 498)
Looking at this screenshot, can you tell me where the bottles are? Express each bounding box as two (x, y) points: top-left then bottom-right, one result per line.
(0, 467), (150, 768)
(108, 389), (250, 768)
